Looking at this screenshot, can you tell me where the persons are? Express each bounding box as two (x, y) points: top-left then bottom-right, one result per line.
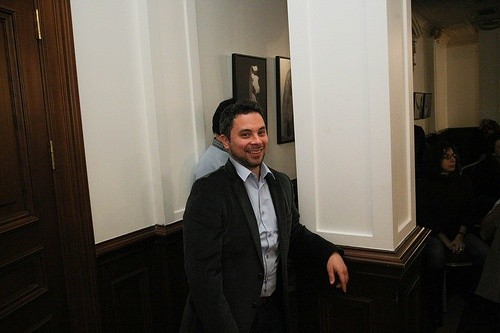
(180, 99), (349, 333)
(416, 143), (490, 330)
(477, 119), (500, 161)
(475, 132), (500, 218)
(194, 98), (242, 181)
(474, 198), (500, 333)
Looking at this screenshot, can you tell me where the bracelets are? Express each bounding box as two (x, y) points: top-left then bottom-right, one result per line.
(458, 232), (465, 236)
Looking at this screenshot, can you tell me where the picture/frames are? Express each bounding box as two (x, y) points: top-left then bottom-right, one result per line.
(231, 53), (267, 135)
(275, 56), (295, 145)
(413, 92), (433, 120)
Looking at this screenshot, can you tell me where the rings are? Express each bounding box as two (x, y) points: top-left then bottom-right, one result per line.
(460, 249), (463, 251)
